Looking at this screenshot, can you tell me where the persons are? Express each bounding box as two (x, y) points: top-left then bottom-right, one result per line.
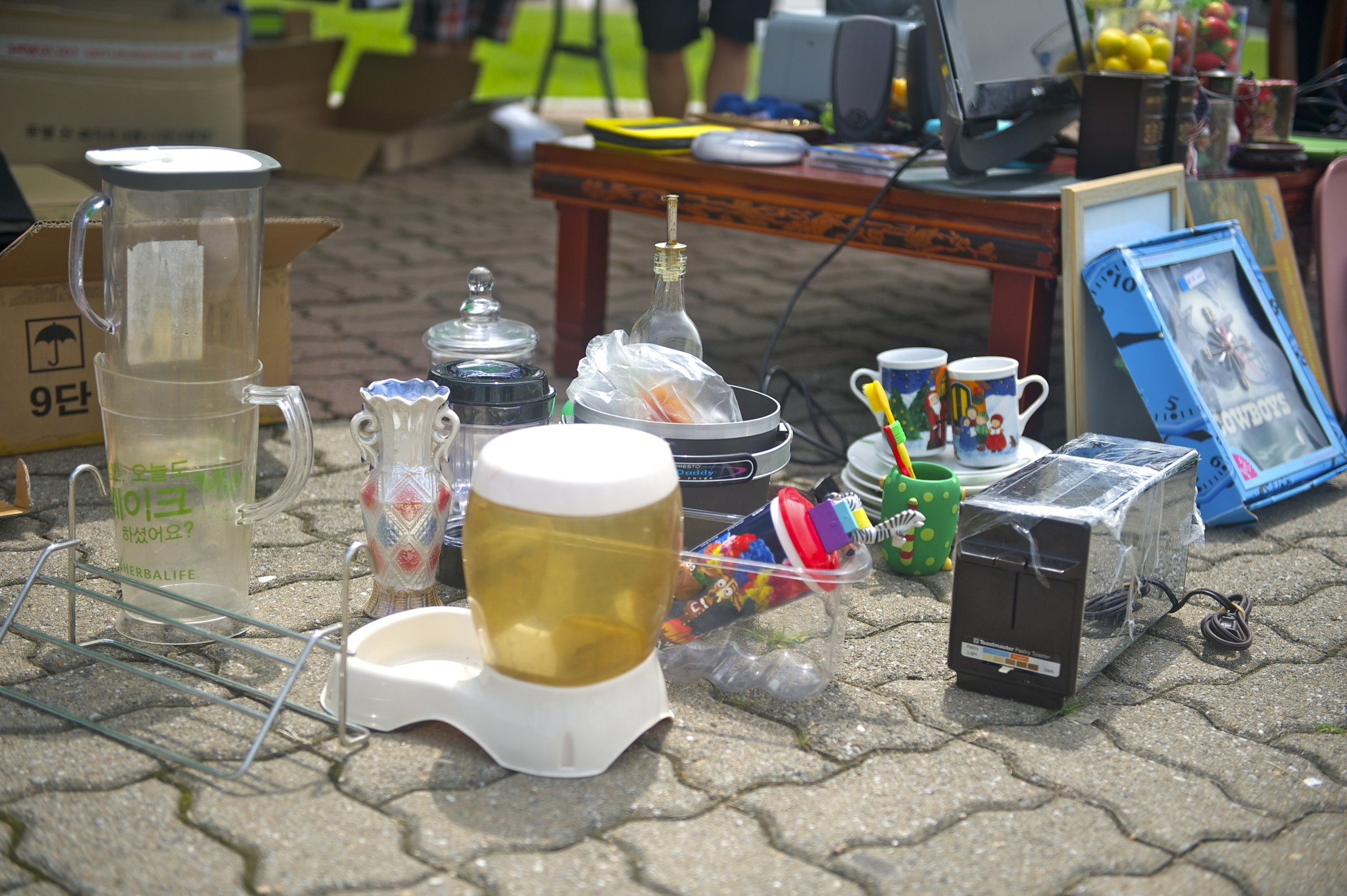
(409, 0), (516, 58)
(632, 0), (772, 118)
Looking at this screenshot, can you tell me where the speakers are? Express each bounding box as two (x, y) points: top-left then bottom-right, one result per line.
(756, 10), (934, 144)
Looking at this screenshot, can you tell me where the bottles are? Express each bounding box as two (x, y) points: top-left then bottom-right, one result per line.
(422, 266), (540, 515)
(351, 377), (461, 618)
(630, 194), (703, 361)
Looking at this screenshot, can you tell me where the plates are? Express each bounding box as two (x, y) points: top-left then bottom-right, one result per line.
(840, 425), (1052, 524)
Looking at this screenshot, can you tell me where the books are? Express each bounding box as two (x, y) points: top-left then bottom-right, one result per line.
(811, 144), (947, 179)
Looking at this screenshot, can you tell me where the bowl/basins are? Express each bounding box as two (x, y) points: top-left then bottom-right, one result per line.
(657, 508), (873, 701)
(1092, 1), (1249, 75)
(1032, 14), (1095, 94)
(1230, 142), (1307, 173)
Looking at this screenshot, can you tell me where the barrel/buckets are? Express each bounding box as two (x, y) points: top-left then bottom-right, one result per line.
(561, 384), (794, 551)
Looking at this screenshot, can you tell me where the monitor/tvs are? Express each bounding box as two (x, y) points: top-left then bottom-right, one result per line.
(915, 0), (1095, 177)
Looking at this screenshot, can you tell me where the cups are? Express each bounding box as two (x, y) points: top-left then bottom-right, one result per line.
(68, 146), (281, 474)
(946, 356), (1049, 468)
(880, 461), (966, 575)
(93, 352), (315, 645)
(462, 423), (684, 686)
(428, 359), (556, 548)
(849, 347), (948, 457)
(658, 487), (926, 652)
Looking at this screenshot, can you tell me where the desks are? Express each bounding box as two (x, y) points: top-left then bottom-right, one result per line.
(532, 134), (1323, 444)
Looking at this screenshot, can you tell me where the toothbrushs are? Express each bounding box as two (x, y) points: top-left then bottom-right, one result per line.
(883, 420), (911, 477)
(862, 381), (917, 479)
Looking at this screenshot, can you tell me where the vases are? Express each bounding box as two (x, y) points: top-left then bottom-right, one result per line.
(351, 373), (460, 618)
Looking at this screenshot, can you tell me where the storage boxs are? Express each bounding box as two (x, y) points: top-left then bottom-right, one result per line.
(1081, 218), (1347, 527)
(0, 0), (497, 460)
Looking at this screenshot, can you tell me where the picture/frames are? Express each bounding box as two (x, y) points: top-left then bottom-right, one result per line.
(1060, 161), (1188, 444)
